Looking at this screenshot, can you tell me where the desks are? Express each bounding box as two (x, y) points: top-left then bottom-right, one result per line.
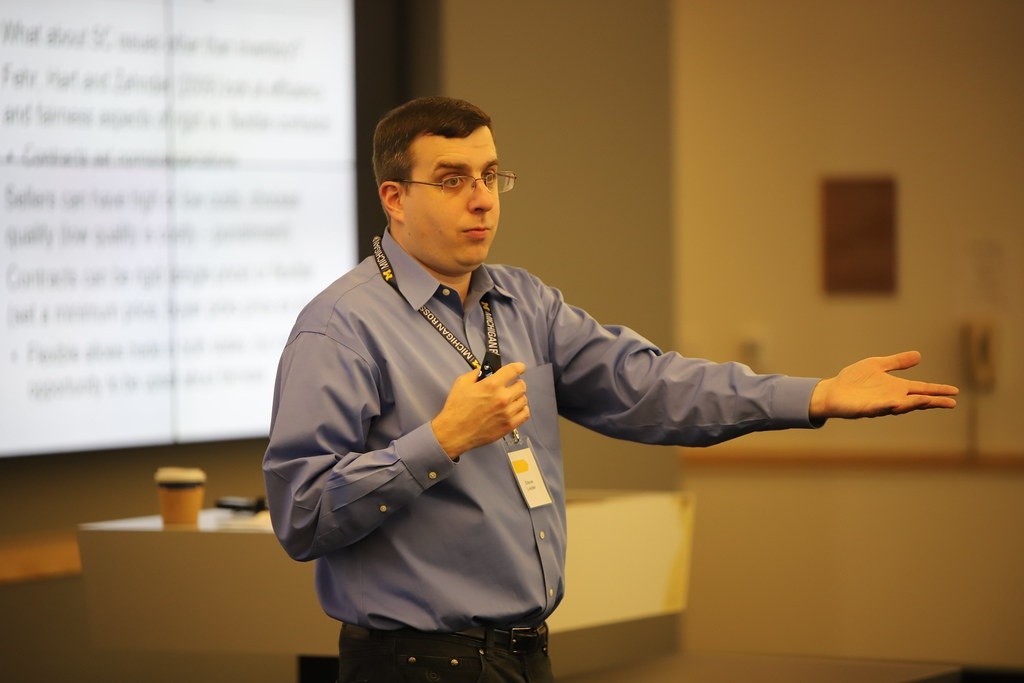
(77, 489), (697, 683)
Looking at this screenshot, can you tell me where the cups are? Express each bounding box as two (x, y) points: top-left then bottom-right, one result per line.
(153, 467), (207, 525)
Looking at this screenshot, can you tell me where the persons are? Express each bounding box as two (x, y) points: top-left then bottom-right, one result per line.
(262, 97), (958, 683)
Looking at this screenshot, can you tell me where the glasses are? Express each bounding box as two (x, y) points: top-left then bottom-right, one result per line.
(398, 169), (519, 198)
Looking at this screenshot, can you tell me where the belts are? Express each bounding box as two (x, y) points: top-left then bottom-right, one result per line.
(345, 613), (550, 657)
